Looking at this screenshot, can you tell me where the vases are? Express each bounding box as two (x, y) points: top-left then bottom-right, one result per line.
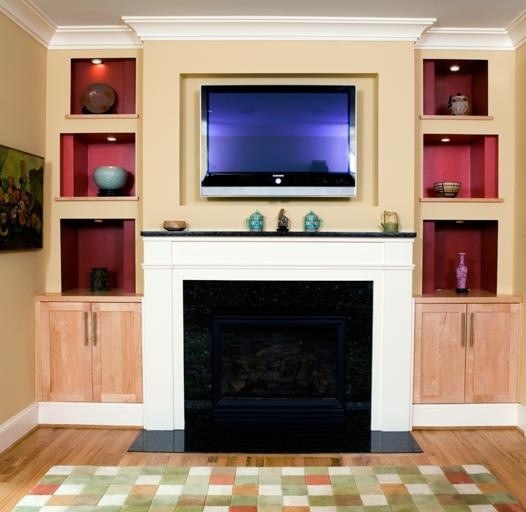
(456, 251), (469, 288)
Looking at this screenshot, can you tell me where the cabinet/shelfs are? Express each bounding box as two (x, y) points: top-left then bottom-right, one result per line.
(419, 59), (503, 306)
(52, 57), (140, 300)
(34, 296), (144, 404)
(416, 300), (516, 405)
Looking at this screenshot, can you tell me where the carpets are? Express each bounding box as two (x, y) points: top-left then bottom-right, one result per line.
(11, 465), (526, 512)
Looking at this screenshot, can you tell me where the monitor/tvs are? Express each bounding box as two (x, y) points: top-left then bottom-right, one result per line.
(200, 84), (357, 197)
